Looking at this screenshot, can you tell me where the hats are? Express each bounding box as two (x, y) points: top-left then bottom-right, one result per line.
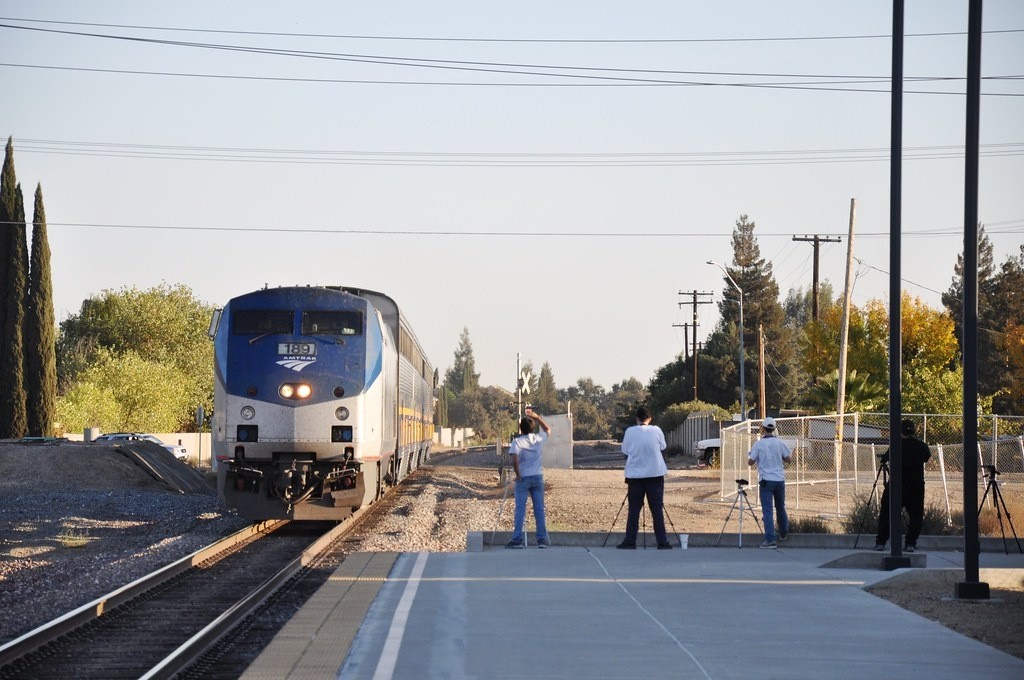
(762, 417), (776, 428)
(901, 419), (915, 435)
(637, 407), (650, 418)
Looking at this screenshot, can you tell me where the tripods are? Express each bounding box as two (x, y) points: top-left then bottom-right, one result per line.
(602, 493), (682, 549)
(979, 476), (1023, 555)
(714, 483), (763, 548)
(853, 460), (908, 549)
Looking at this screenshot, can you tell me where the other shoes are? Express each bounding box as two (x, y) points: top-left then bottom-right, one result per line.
(657, 542), (673, 549)
(505, 539), (523, 548)
(616, 541), (635, 549)
(874, 545), (884, 551)
(904, 546), (913, 551)
(537, 539), (547, 547)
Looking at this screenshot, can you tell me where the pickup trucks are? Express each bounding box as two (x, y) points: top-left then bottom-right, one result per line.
(695, 426), (815, 470)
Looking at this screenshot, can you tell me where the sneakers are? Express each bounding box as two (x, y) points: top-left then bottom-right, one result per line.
(759, 541), (777, 549)
(779, 534), (787, 540)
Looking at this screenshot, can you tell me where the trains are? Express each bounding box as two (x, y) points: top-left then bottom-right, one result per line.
(209, 284), (435, 521)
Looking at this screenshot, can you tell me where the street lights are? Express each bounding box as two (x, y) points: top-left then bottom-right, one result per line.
(706, 260), (746, 423)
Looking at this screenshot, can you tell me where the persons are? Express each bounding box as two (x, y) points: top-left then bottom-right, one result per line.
(747, 417), (792, 549)
(504, 408), (552, 550)
(874, 420), (932, 551)
(615, 408), (673, 549)
